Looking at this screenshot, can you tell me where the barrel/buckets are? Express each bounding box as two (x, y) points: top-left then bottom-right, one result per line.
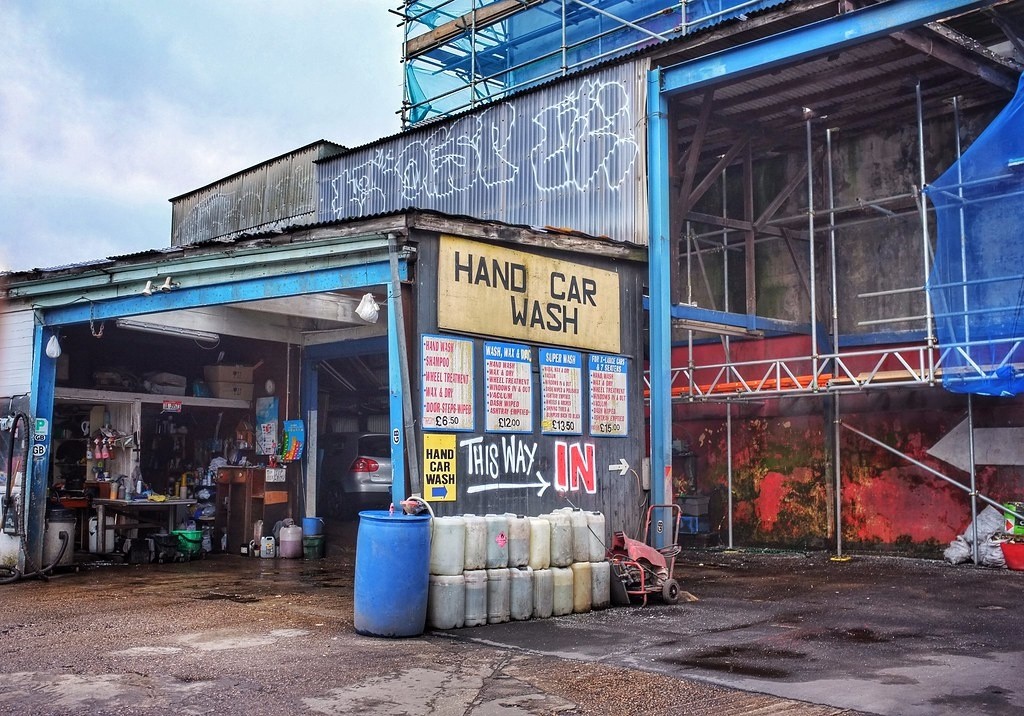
(429, 507), (611, 630)
(353, 510), (432, 638)
(239, 543), (249, 556)
(260, 535), (275, 558)
(303, 537), (324, 560)
(301, 517), (323, 536)
(279, 525), (302, 559)
(171, 529), (203, 559)
(88, 515), (115, 553)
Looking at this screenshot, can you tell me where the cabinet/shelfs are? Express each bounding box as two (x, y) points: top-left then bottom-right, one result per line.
(213, 467), (283, 554)
(52, 438), (98, 485)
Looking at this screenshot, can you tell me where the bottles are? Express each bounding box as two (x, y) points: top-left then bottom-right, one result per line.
(207, 473), (211, 486)
(110, 482), (118, 499)
(679, 491), (686, 498)
(118, 484), (125, 499)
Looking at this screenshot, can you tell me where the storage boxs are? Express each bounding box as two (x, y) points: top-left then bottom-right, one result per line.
(207, 382), (254, 400)
(673, 514), (709, 534)
(204, 366), (254, 383)
(678, 497), (709, 516)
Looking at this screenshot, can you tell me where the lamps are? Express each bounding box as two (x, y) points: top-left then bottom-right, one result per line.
(671, 318), (765, 339)
(116, 319), (219, 342)
(141, 281), (157, 295)
(161, 276), (180, 292)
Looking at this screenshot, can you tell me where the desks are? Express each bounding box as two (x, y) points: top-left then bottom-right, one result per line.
(90, 499), (197, 554)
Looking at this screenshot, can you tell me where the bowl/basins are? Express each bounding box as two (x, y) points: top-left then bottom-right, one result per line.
(1001, 542), (1024, 571)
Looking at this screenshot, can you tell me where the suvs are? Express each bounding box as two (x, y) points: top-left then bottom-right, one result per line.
(320, 430), (392, 524)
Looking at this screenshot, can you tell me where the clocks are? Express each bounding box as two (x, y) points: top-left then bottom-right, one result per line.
(265, 379), (275, 394)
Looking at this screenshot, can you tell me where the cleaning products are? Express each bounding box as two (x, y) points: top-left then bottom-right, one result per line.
(93, 437), (116, 460)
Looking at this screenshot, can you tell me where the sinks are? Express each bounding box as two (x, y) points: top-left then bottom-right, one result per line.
(215, 466), (258, 484)
